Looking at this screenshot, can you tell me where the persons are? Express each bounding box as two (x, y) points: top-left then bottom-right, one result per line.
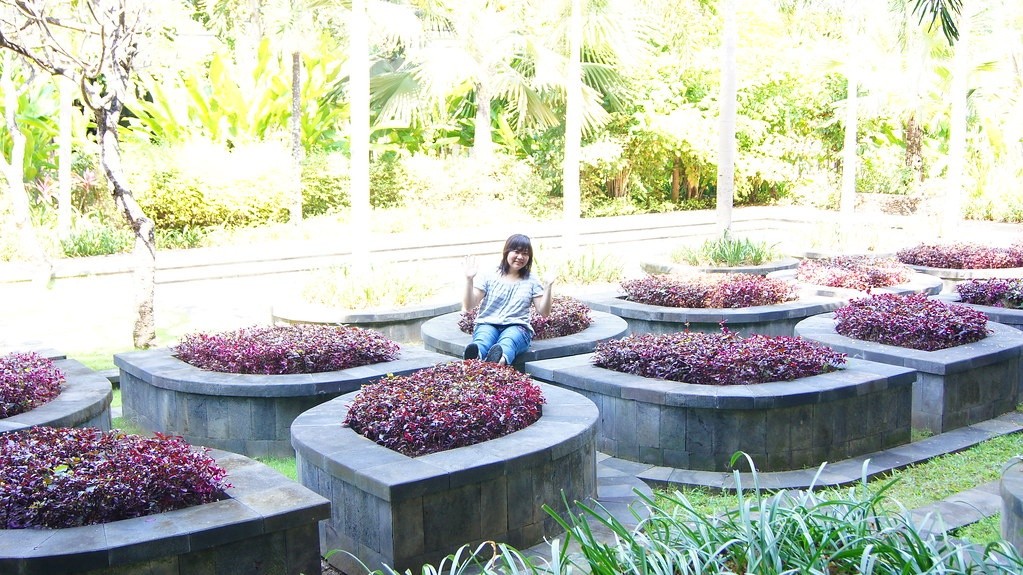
(464, 234), (558, 364)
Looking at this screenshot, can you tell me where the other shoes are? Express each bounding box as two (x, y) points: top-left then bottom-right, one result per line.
(464, 344), (478, 358)
(484, 344), (503, 364)
